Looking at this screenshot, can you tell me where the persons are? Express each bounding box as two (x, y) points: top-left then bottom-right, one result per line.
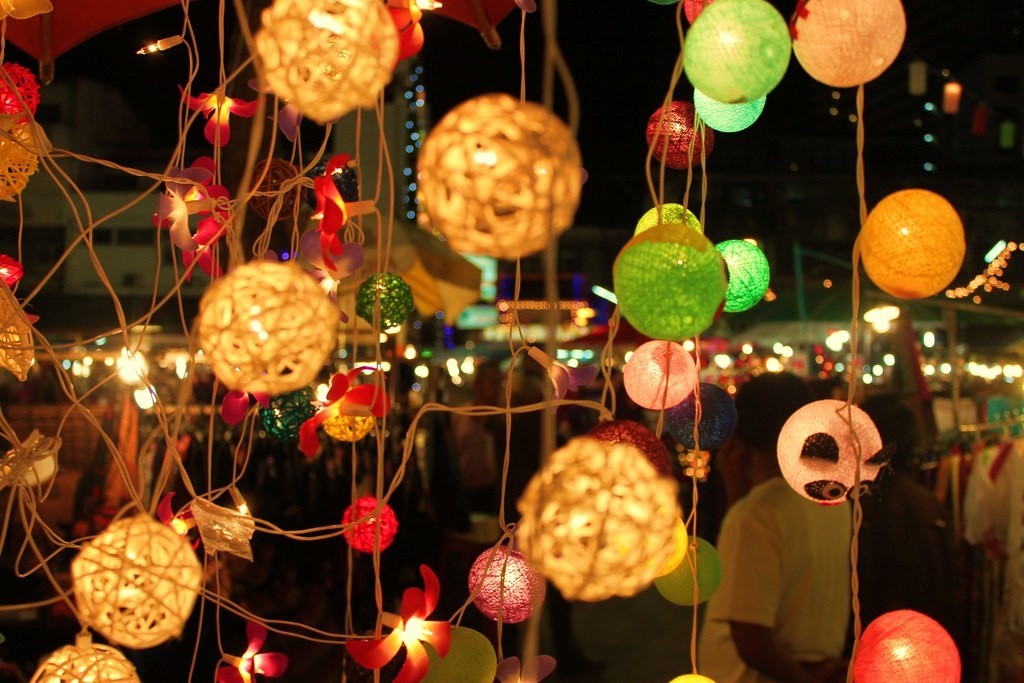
(66, 346), (1013, 683)
